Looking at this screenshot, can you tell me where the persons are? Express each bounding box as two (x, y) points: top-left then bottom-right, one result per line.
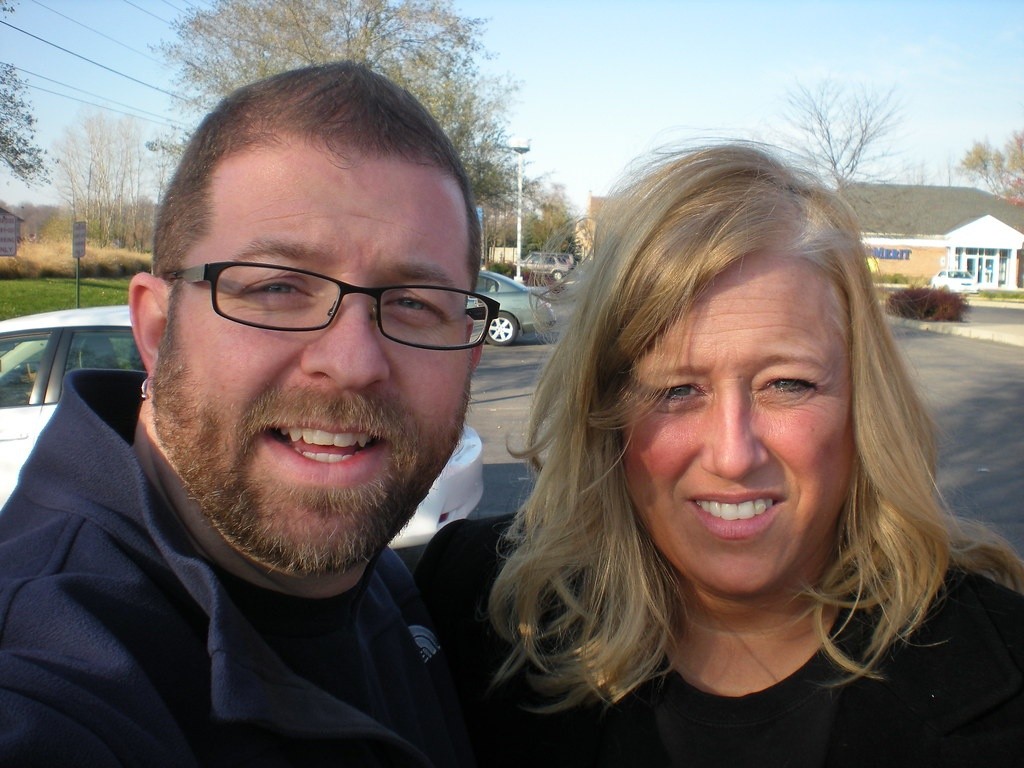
(412, 139), (1024, 768)
(1, 62), (477, 768)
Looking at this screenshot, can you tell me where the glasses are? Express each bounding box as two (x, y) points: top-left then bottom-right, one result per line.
(162, 261), (500, 351)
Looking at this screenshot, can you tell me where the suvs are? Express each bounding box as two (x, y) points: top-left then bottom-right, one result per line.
(515, 252), (579, 281)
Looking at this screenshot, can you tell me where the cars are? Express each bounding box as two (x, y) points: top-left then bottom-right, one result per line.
(465, 270), (558, 346)
(0, 304), (485, 552)
(930, 269), (981, 296)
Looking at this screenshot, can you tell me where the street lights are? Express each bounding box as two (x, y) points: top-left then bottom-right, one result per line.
(512, 146), (529, 283)
